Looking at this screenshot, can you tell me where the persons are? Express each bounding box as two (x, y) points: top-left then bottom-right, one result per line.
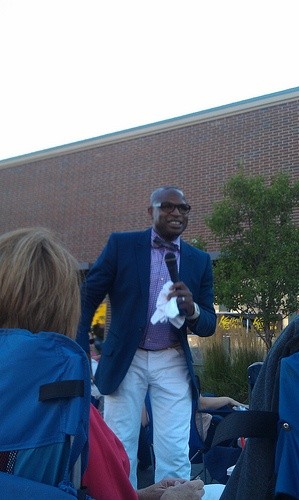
(76, 187), (218, 491)
(0, 229), (248, 500)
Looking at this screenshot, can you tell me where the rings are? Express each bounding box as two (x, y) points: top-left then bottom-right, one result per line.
(182, 297), (185, 302)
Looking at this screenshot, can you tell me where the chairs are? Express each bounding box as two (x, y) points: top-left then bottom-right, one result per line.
(144, 374), (238, 485)
(0, 327), (93, 500)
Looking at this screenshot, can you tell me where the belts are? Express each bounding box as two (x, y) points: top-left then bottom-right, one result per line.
(138, 345), (181, 351)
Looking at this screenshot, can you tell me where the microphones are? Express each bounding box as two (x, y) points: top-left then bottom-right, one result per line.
(164, 252), (186, 317)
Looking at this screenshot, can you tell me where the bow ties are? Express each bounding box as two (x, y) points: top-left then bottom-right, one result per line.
(153, 237), (179, 252)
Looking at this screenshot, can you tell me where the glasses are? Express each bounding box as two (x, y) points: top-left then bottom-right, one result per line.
(151, 201), (191, 215)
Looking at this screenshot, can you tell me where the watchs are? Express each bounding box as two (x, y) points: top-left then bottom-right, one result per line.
(184, 301), (201, 321)
